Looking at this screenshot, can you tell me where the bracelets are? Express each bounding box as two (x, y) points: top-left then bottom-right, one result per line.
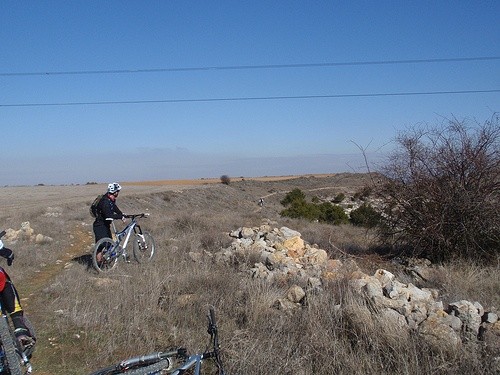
(122, 217), (125, 220)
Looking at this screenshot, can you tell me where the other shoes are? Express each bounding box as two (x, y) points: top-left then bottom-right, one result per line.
(14, 327), (36, 345)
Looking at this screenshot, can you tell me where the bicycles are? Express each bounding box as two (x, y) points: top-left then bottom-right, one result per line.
(88, 306), (224, 375)
(0, 230), (35, 375)
(91, 213), (155, 273)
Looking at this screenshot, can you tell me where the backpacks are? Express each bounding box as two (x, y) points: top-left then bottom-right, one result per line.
(89, 195), (113, 217)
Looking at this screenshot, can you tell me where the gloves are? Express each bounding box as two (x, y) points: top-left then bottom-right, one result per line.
(0, 247), (14, 266)
(122, 216), (126, 223)
(122, 213), (127, 217)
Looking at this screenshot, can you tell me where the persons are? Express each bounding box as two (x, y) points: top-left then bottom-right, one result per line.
(259, 198), (264, 206)
(0, 239), (36, 375)
(92, 182), (129, 270)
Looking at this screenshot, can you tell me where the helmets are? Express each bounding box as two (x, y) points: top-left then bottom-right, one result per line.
(108, 183), (122, 194)
